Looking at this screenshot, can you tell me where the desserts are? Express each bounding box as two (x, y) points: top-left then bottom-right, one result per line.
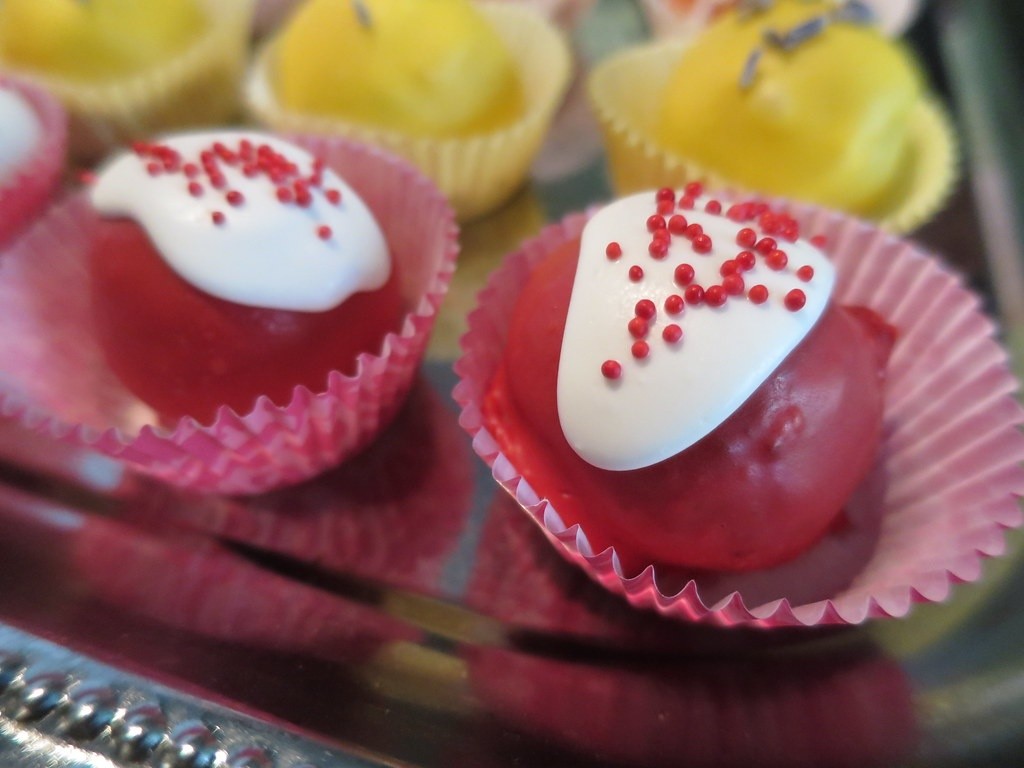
(0, 0), (1024, 631)
